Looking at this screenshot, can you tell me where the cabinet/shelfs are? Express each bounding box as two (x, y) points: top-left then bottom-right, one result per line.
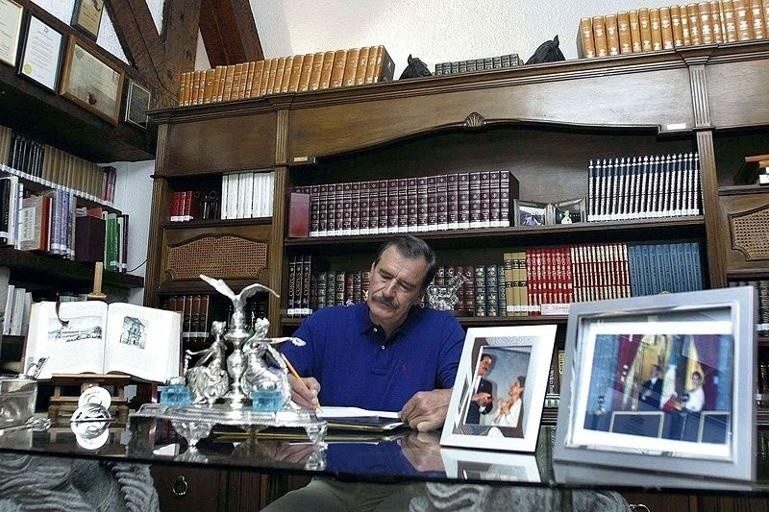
(0, 163), (145, 418)
(135, 38), (769, 512)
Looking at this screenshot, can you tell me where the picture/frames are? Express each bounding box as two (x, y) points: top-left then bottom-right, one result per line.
(122, 77), (152, 134)
(552, 284), (759, 483)
(0, 0), (26, 69)
(70, 0), (106, 42)
(438, 446), (541, 483)
(438, 324), (559, 454)
(59, 34), (126, 127)
(17, 8), (67, 96)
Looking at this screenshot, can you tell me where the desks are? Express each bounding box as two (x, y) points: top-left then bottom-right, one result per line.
(0, 419), (768, 512)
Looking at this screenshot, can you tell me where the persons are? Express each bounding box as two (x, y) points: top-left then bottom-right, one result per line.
(561, 209), (574, 224)
(634, 363), (664, 413)
(685, 371), (703, 414)
(491, 376), (526, 429)
(463, 353), (494, 425)
(260, 231), (467, 512)
(522, 209), (543, 226)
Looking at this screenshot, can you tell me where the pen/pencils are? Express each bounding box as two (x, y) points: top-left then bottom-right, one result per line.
(279, 351), (325, 413)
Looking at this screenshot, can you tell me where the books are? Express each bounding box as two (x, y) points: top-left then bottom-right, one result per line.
(177, 44), (397, 108)
(0, 174), (131, 274)
(167, 188), (198, 220)
(286, 170), (521, 239)
(20, 301), (184, 391)
(0, 266), (36, 336)
(545, 342), (569, 409)
(286, 240), (705, 324)
(578, 0), (769, 60)
(583, 152), (705, 222)
(1, 124), (118, 203)
(435, 53), (525, 83)
(219, 169), (275, 220)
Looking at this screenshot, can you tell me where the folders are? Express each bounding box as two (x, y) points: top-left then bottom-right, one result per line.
(316, 405), (408, 431)
(213, 416), (407, 441)
